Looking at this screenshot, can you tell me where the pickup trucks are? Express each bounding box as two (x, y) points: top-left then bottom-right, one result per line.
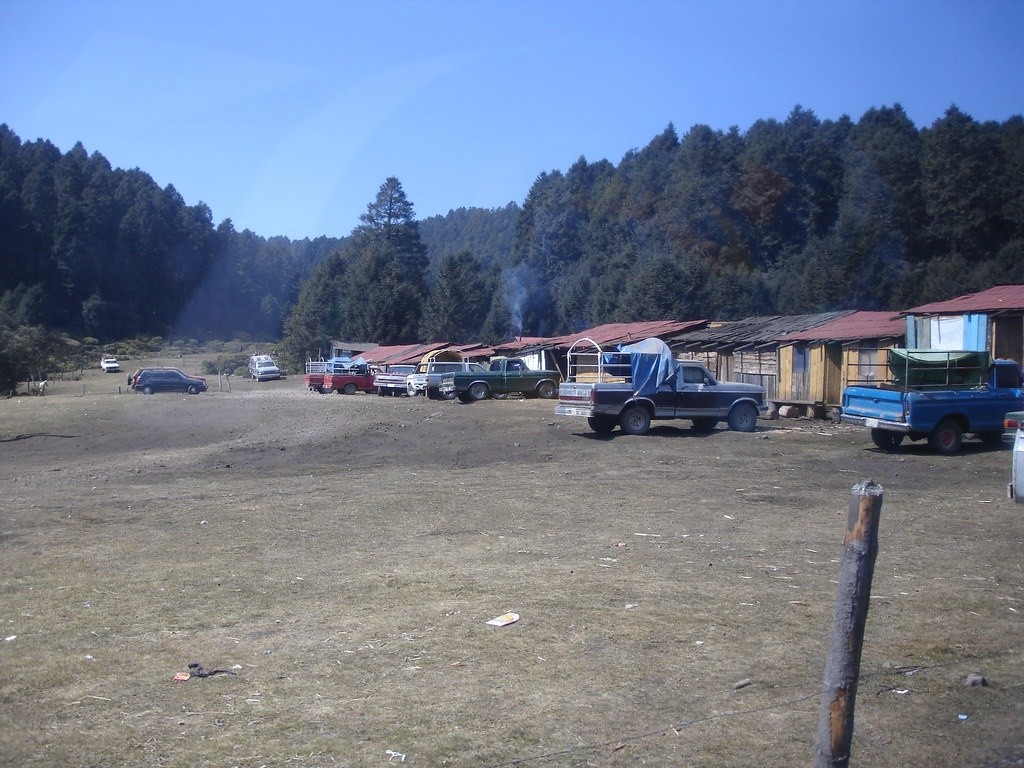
(438, 358), (560, 402)
(101, 357), (120, 372)
(1001, 411), (1024, 505)
(304, 372), (325, 391)
(249, 356), (281, 382)
(413, 362), (489, 400)
(554, 359), (769, 435)
(839, 359), (1024, 452)
(322, 365), (384, 396)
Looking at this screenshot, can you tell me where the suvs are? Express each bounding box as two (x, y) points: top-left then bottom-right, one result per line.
(127, 367), (208, 395)
(373, 365), (416, 396)
